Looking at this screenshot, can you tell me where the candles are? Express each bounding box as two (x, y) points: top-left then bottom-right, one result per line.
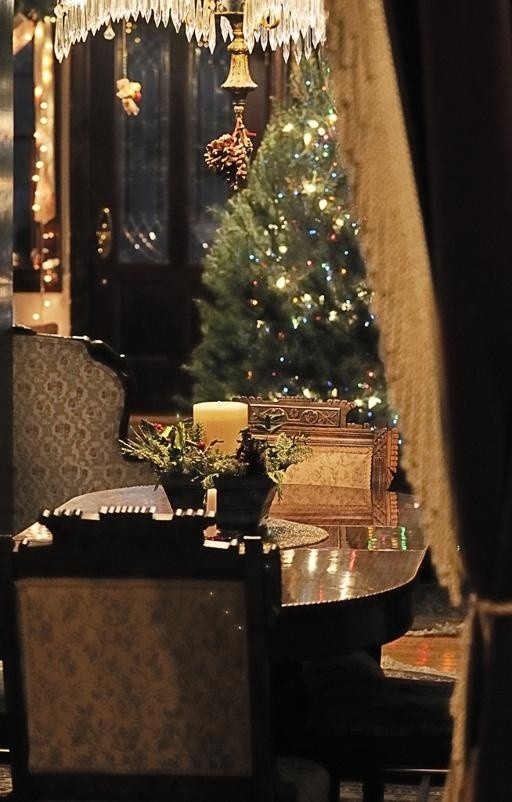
(191, 399), (249, 460)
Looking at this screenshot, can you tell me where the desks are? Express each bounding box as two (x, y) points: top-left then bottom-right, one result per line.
(13, 482), (432, 800)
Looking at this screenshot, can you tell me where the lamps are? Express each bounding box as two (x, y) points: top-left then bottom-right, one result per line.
(53, 0), (327, 192)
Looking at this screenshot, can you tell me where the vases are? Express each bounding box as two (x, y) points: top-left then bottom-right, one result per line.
(213, 473), (281, 527)
(156, 468), (209, 512)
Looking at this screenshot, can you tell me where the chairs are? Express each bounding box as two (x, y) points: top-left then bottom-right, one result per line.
(227, 393), (400, 527)
(0, 324), (160, 537)
(381, 621), (461, 774)
(0, 503), (331, 801)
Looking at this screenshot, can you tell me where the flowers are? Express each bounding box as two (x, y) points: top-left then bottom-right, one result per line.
(120, 418), (310, 494)
